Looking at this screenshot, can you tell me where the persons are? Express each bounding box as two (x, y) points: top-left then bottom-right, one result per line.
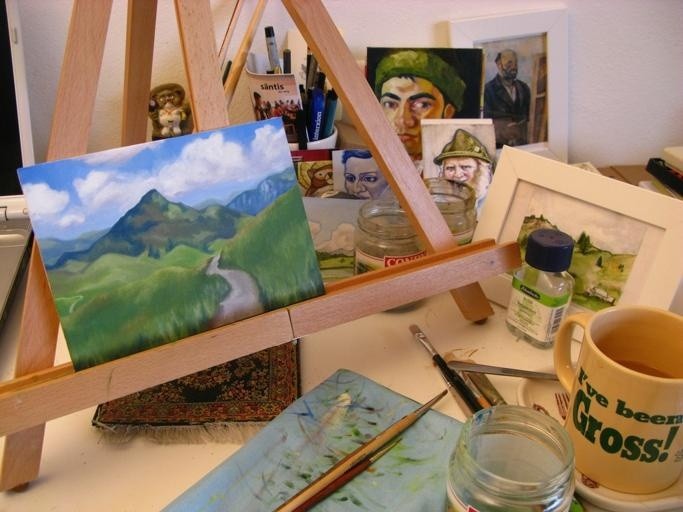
(373, 49), (466, 163)
(252, 91), (300, 124)
(342, 151), (389, 199)
(433, 128), (497, 218)
(484, 49), (530, 148)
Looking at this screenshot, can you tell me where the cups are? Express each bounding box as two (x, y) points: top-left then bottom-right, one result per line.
(553, 306), (683, 494)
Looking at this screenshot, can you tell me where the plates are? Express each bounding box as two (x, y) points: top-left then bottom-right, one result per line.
(516, 360), (682, 512)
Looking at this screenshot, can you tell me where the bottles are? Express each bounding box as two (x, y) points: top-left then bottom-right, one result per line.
(353, 178), (477, 310)
(446, 405), (574, 512)
(506, 228), (576, 349)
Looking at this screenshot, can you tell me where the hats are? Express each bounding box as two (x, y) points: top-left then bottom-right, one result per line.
(433, 128), (492, 165)
(374, 50), (466, 112)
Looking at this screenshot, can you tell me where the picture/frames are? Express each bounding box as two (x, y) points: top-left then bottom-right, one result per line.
(451, 5), (571, 167)
(466, 143), (683, 344)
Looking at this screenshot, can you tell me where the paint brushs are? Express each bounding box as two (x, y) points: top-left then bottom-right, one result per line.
(274, 389), (448, 512)
(433, 353), (507, 410)
(409, 324), (484, 413)
(292, 437), (403, 512)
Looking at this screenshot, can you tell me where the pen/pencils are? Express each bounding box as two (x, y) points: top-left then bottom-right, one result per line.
(264, 26), (283, 75)
(246, 53), (257, 73)
(283, 49), (292, 74)
(299, 47), (338, 141)
(296, 111), (307, 150)
